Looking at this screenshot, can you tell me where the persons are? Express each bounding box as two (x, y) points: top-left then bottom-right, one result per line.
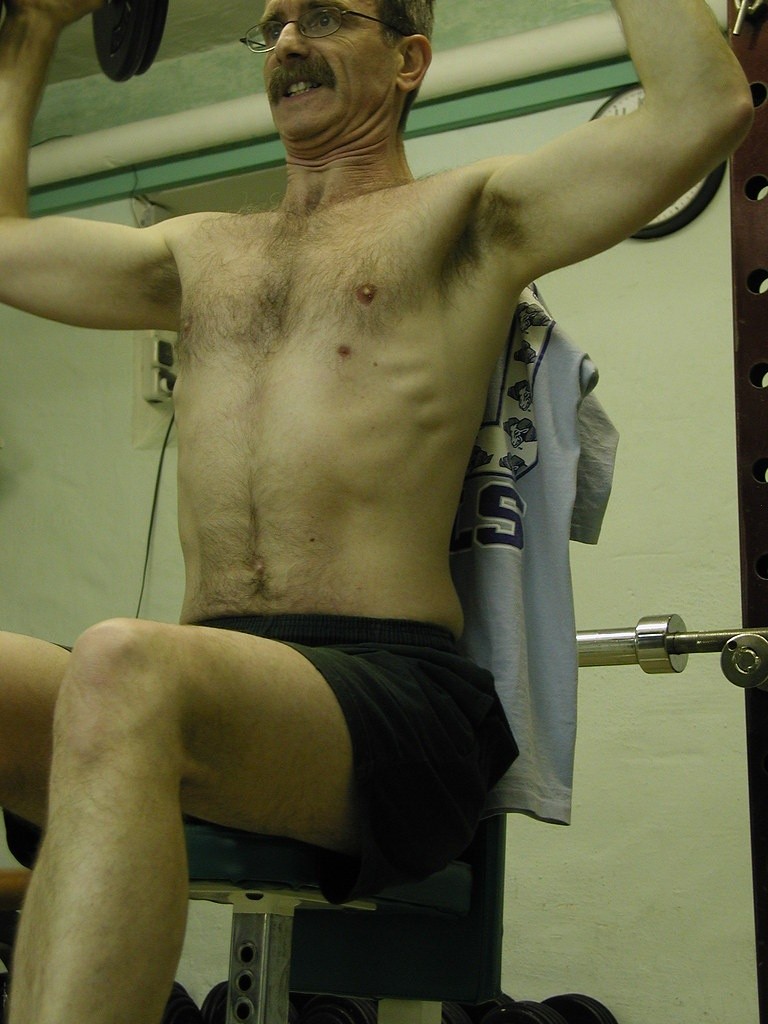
(1, 0), (761, 1024)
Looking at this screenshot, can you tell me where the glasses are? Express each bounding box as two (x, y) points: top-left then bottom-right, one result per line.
(240, 7), (408, 53)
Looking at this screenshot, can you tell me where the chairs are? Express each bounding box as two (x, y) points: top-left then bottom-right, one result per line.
(181, 280), (622, 1024)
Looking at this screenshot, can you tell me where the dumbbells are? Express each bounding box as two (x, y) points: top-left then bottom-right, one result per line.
(0, 0), (170, 83)
